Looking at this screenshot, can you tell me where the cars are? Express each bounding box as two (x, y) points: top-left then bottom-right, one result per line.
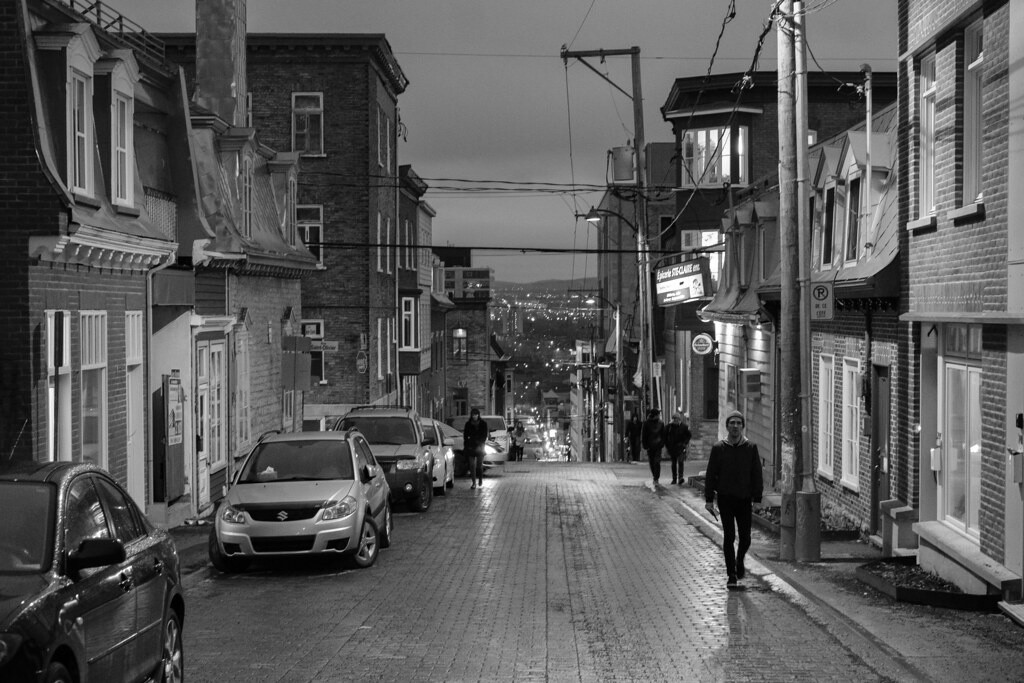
(452, 414), (515, 465)
(0, 461), (188, 683)
(420, 417), (456, 496)
(433, 419), (507, 478)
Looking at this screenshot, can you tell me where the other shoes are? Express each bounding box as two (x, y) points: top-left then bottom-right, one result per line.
(479, 479), (482, 486)
(654, 480), (658, 487)
(735, 559), (745, 579)
(727, 575), (738, 586)
(471, 484), (476, 489)
(679, 478), (684, 484)
(671, 479), (676, 484)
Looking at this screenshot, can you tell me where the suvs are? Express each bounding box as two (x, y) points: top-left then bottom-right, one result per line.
(332, 404), (438, 512)
(208, 426), (394, 569)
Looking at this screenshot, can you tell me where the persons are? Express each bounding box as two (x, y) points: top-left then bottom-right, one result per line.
(512, 419), (526, 462)
(704, 411), (764, 587)
(623, 408), (692, 487)
(463, 408), (487, 489)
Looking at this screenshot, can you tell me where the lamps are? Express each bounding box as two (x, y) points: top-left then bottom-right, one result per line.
(585, 205), (638, 234)
(585, 293), (617, 312)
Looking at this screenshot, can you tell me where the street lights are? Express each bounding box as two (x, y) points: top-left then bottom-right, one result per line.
(582, 205), (654, 411)
(585, 294), (624, 462)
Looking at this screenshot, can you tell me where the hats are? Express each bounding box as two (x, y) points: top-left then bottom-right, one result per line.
(725, 410), (745, 429)
(672, 413), (682, 421)
(649, 409), (660, 417)
